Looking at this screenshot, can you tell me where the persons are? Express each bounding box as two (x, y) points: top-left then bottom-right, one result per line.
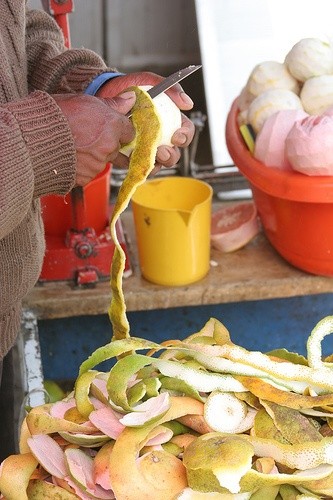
(1, 0), (194, 460)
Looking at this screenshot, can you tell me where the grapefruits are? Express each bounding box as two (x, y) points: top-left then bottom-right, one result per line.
(211, 202), (259, 252)
(252, 110), (333, 176)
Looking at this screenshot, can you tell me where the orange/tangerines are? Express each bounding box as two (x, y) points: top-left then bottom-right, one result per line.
(236, 38), (333, 135)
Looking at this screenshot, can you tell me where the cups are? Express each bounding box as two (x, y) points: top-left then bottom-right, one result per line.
(130, 174), (215, 287)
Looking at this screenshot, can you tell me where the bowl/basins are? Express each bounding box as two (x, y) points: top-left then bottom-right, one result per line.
(223, 83), (332, 278)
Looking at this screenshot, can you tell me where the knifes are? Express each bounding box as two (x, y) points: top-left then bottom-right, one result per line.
(123, 65), (203, 120)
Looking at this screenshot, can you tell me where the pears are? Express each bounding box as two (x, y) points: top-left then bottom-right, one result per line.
(118, 84), (183, 161)
